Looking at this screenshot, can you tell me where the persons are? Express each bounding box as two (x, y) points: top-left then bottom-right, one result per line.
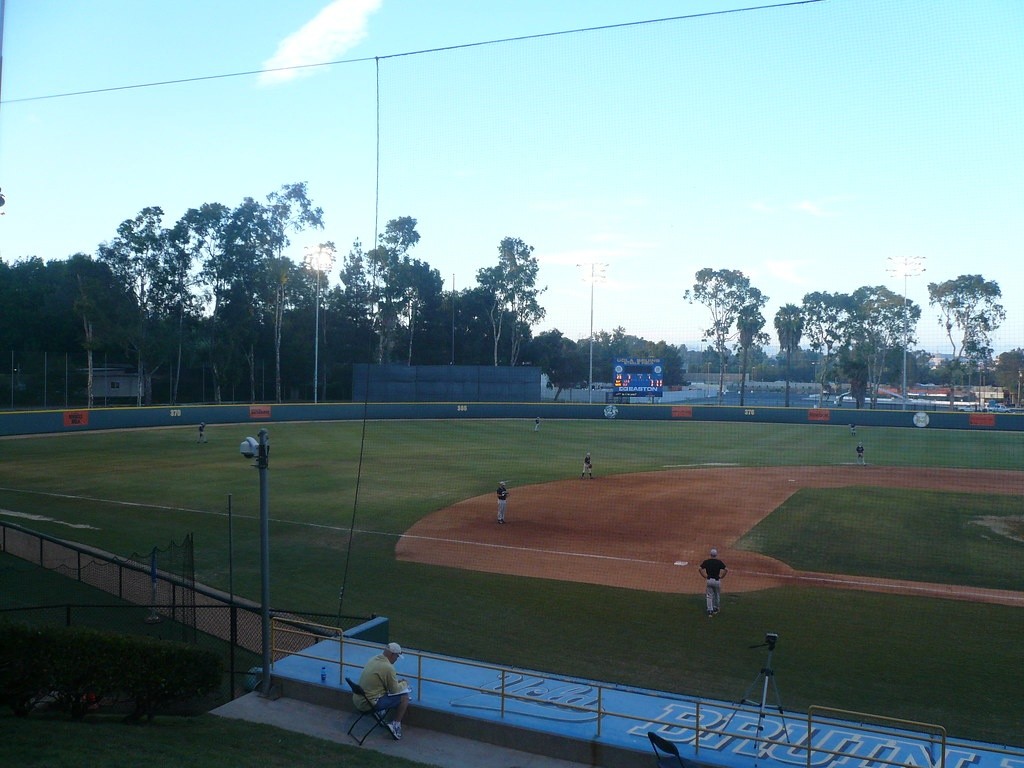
(197, 422), (208, 443)
(856, 442), (865, 466)
(496, 482), (509, 524)
(534, 417), (539, 431)
(699, 549), (728, 617)
(353, 643), (413, 740)
(851, 424), (855, 436)
(581, 453), (594, 479)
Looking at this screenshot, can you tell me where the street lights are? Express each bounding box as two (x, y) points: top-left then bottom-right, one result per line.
(886, 254), (927, 410)
(576, 262), (610, 404)
(738, 365), (742, 390)
(239, 429), (271, 697)
(706, 362), (712, 396)
(812, 362), (820, 407)
(304, 243), (330, 405)
(1017, 369), (1023, 408)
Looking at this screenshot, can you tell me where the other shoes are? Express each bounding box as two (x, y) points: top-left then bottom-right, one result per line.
(709, 612), (713, 618)
(498, 519), (505, 524)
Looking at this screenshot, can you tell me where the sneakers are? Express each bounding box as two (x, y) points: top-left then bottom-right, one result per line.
(387, 722), (402, 740)
(375, 709), (387, 727)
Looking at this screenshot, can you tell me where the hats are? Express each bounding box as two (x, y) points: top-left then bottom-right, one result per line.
(500, 482), (506, 486)
(711, 549), (717, 556)
(386, 642), (405, 659)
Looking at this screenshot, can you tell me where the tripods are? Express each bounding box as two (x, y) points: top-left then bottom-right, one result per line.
(719, 643), (791, 768)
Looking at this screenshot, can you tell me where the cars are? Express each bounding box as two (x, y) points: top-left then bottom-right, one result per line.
(985, 404), (1011, 413)
(959, 403), (979, 412)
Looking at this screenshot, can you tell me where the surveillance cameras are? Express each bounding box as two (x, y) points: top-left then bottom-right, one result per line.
(241, 438), (256, 458)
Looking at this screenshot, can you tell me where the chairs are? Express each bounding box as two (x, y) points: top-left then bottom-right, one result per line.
(345, 678), (391, 746)
(647, 732), (699, 768)
